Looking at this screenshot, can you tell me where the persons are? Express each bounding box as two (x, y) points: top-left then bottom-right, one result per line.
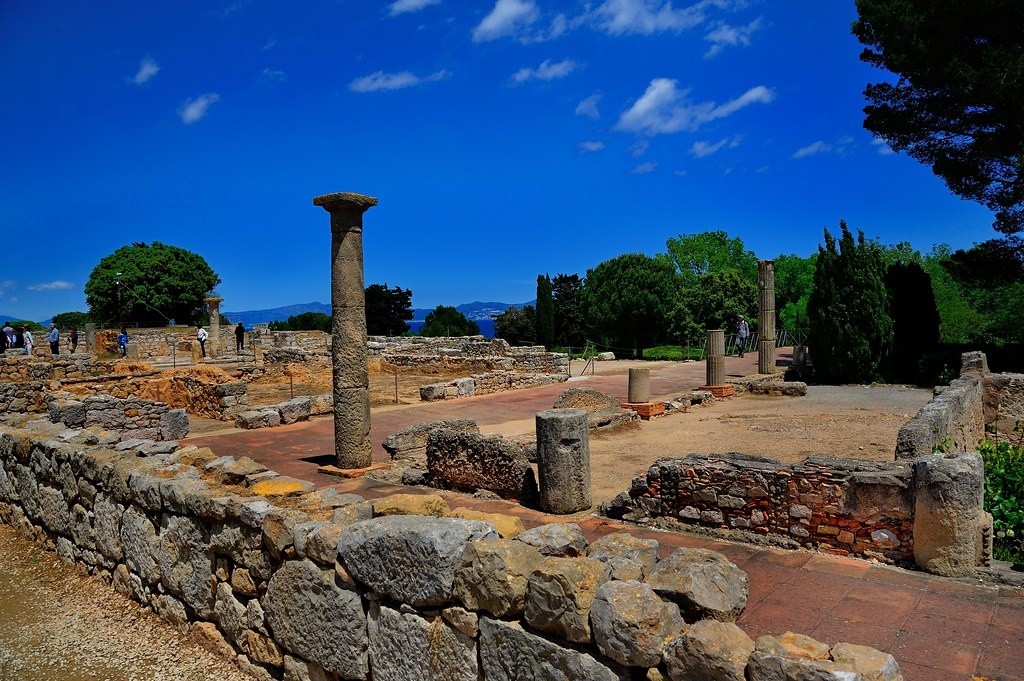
(48, 323), (60, 355)
(67, 328), (78, 354)
(736, 315), (749, 358)
(234, 322), (245, 353)
(117, 328), (128, 357)
(197, 324), (208, 357)
(0, 321), (33, 355)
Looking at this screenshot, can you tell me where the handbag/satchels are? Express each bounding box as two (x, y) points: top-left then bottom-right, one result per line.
(197, 337), (202, 342)
(67, 337), (73, 350)
